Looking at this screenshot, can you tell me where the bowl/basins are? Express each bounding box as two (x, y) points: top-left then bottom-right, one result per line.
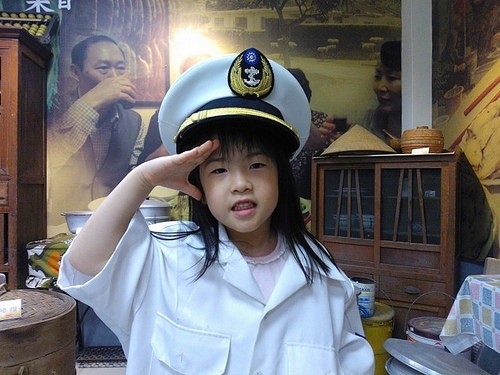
(61, 210), (93, 233)
(332, 214), (374, 231)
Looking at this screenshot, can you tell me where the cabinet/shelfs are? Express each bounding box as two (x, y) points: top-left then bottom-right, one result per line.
(308, 154), (460, 313)
(0, 26), (54, 293)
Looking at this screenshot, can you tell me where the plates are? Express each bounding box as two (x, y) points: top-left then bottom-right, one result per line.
(332, 187), (369, 194)
(148, 221), (200, 240)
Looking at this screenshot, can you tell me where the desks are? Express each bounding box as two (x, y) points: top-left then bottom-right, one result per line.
(439, 274), (500, 367)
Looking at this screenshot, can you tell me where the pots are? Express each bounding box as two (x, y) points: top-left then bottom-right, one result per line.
(140, 197), (177, 224)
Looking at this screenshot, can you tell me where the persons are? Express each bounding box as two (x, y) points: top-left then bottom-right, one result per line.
(45, 34), (477, 228)
(57, 47), (375, 375)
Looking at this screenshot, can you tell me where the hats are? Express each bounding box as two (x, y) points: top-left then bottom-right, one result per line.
(319, 124), (397, 155)
(157, 47), (310, 163)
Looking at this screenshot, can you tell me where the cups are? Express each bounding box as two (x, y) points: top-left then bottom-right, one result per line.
(118, 98), (135, 110)
(331, 115), (347, 135)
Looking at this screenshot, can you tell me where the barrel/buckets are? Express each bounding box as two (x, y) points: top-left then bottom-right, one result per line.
(360, 286), (395, 375)
(349, 276), (376, 318)
(406, 290), (471, 362)
